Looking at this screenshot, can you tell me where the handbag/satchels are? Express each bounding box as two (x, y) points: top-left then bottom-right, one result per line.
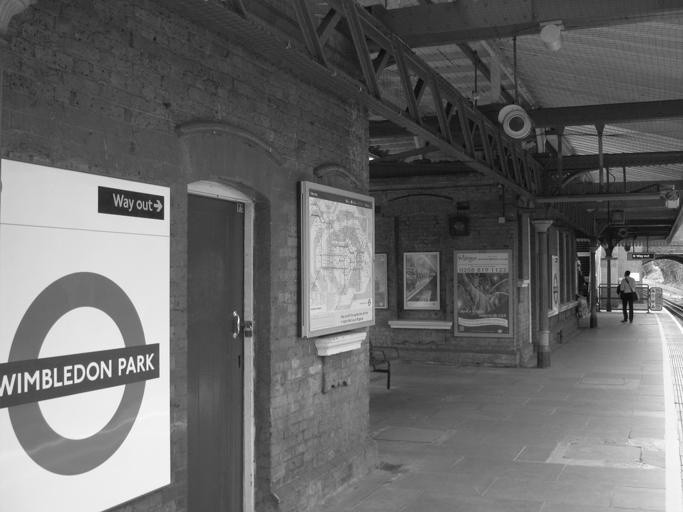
(632, 292), (639, 301)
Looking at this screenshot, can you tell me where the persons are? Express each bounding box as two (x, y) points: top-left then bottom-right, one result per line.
(619, 271), (635, 323)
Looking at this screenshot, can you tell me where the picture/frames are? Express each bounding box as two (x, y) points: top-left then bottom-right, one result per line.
(452, 248), (514, 340)
(374, 252), (388, 310)
(402, 250), (441, 311)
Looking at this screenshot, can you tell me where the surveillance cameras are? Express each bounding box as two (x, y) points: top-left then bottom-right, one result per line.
(664, 191), (680, 209)
